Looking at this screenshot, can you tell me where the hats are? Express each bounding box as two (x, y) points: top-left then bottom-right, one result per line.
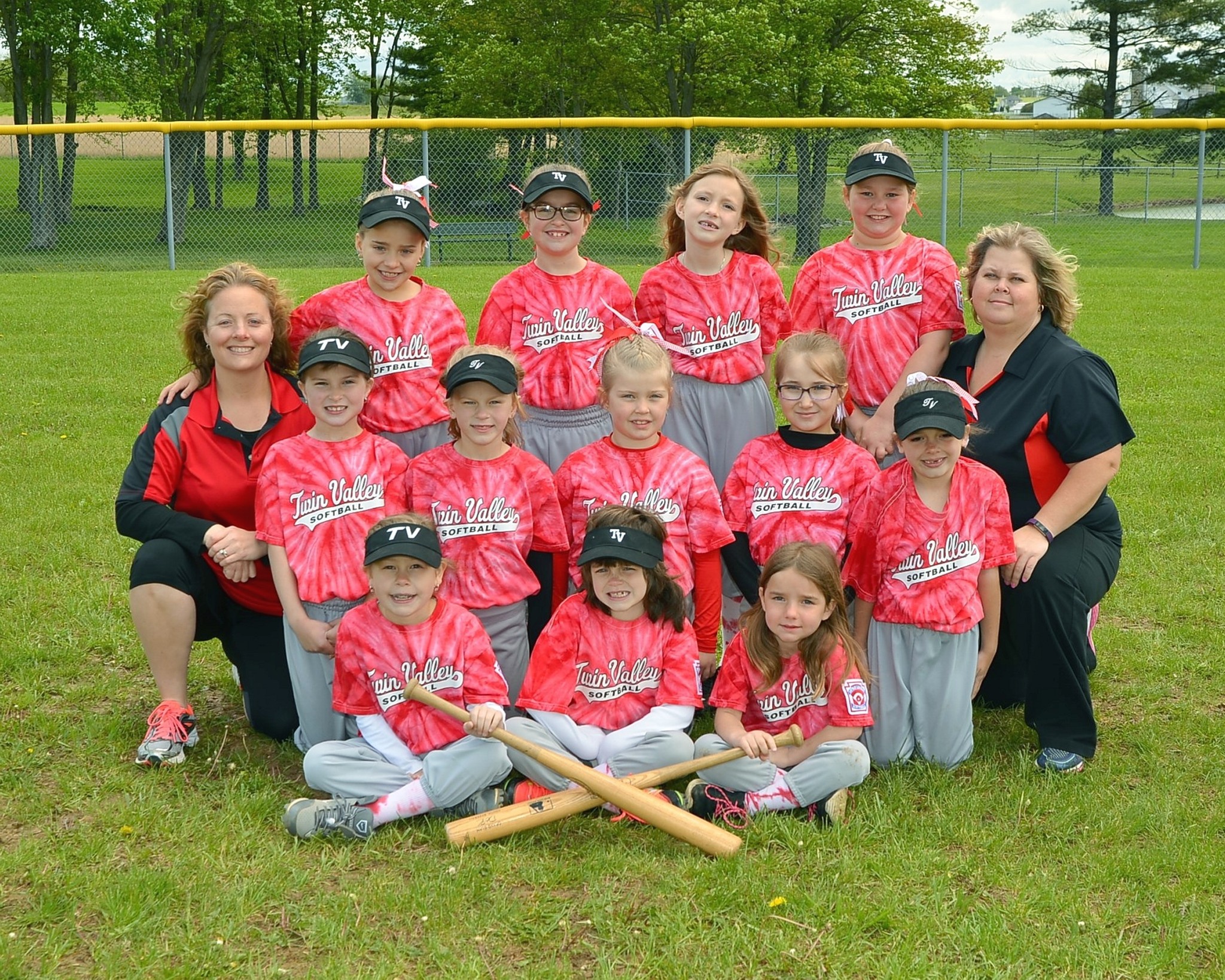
(576, 526), (665, 569)
(523, 171), (593, 214)
(357, 195), (431, 242)
(894, 390), (967, 442)
(297, 336), (371, 378)
(844, 152), (918, 186)
(446, 353), (518, 399)
(362, 523), (443, 570)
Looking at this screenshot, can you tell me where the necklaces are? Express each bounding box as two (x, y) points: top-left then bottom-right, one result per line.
(681, 248), (725, 279)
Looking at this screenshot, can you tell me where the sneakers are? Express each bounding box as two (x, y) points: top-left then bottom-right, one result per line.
(282, 794), (379, 843)
(504, 777), (557, 806)
(807, 788), (848, 826)
(610, 786), (685, 825)
(685, 778), (748, 829)
(134, 698), (200, 767)
(1087, 603), (1100, 656)
(444, 786), (504, 817)
(1035, 747), (1084, 775)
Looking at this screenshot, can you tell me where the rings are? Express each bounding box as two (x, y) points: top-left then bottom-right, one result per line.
(219, 549), (228, 557)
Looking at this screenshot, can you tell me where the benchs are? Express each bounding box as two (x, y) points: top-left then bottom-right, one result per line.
(432, 220), (520, 262)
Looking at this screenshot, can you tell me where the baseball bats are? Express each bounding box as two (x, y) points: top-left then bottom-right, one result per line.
(400, 677), (744, 860)
(445, 723), (805, 848)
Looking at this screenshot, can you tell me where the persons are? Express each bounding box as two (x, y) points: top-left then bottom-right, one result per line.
(840, 373), (1017, 769)
(635, 164), (794, 717)
(405, 343), (570, 723)
(787, 138), (968, 471)
(551, 316), (737, 686)
(157, 157), (471, 460)
(474, 162), (640, 661)
(720, 331), (882, 638)
(504, 505), (705, 823)
(255, 326), (414, 754)
(115, 263), (317, 772)
(684, 538), (872, 828)
(285, 515), (513, 841)
(938, 219), (1136, 778)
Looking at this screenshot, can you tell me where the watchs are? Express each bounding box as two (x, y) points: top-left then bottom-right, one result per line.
(1026, 519), (1054, 544)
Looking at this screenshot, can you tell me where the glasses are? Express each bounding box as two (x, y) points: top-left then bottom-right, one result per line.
(776, 382), (839, 400)
